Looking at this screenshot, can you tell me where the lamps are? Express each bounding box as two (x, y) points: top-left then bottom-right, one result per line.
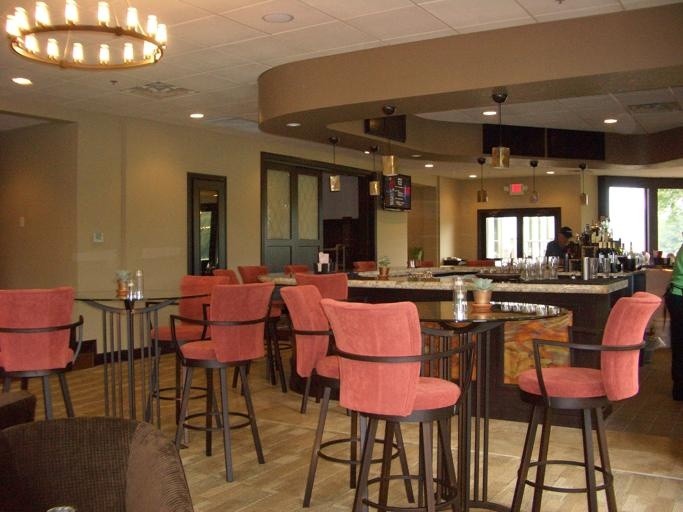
(529, 160), (539, 203)
(579, 163), (588, 206)
(368, 144), (381, 196)
(476, 157), (489, 202)
(381, 104), (399, 177)
(4, 0), (168, 70)
(491, 93), (511, 169)
(328, 136), (341, 192)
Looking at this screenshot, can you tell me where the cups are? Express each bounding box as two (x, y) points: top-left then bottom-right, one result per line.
(116, 266), (144, 298)
(500, 302), (560, 316)
(502, 256), (560, 281)
(449, 277), (471, 311)
(589, 257), (611, 279)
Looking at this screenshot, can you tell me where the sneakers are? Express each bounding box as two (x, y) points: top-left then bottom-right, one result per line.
(672, 382), (682, 401)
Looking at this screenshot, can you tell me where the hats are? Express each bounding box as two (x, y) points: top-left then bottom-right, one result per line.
(560, 226), (573, 238)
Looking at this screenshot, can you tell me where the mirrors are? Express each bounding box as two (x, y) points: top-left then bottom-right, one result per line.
(193, 180), (227, 276)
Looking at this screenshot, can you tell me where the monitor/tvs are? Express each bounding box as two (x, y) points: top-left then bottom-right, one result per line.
(383, 174), (412, 211)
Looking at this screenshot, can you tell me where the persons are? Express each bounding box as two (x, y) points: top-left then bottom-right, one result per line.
(665, 244), (683, 401)
(544, 227), (574, 265)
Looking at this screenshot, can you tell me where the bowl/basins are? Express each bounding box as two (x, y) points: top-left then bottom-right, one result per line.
(653, 256), (672, 265)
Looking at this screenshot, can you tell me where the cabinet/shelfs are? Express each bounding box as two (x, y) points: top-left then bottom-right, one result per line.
(324, 244), (346, 271)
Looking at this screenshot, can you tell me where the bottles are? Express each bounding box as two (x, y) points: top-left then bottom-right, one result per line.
(582, 256), (589, 280)
(622, 241), (651, 271)
(574, 215), (611, 245)
